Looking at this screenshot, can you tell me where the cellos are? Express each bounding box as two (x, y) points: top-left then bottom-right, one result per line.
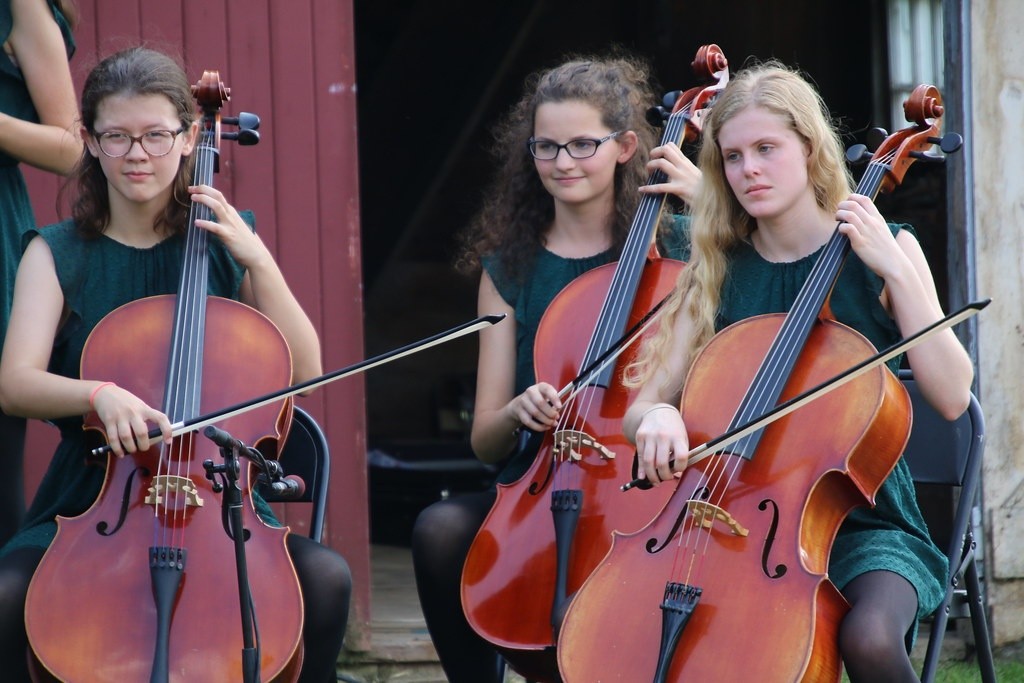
(458, 43), (734, 683)
(18, 65), (308, 683)
(549, 80), (967, 683)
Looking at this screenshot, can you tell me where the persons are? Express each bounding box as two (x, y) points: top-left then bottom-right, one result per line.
(411, 60), (703, 683)
(621, 70), (974, 683)
(0, 47), (354, 683)
(0, 0), (86, 550)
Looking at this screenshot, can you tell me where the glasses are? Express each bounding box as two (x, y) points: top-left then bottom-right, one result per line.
(528, 127), (631, 160)
(92, 123), (187, 158)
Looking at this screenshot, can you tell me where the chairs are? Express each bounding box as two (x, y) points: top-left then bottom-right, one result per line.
(882, 367), (998, 683)
(254, 403), (328, 542)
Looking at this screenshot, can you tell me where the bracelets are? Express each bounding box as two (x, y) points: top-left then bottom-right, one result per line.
(642, 403), (680, 417)
(89, 382), (116, 410)
